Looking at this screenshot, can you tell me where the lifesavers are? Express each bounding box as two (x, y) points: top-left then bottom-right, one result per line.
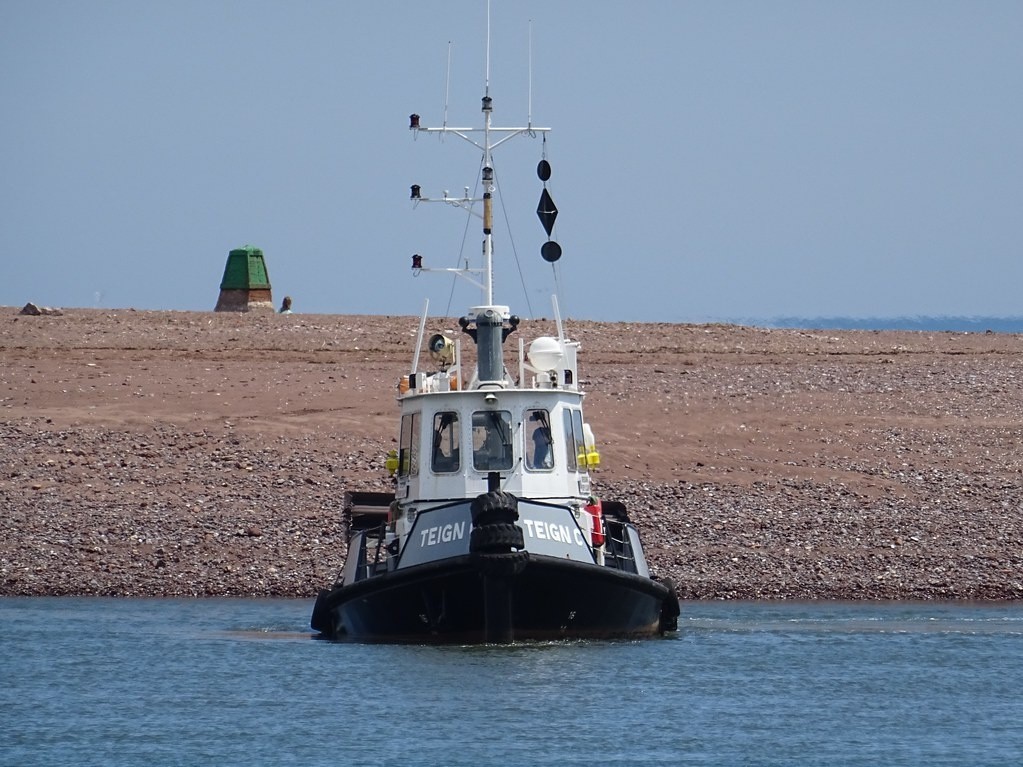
(310, 588), (332, 630)
(469, 490), (532, 577)
(657, 577), (680, 636)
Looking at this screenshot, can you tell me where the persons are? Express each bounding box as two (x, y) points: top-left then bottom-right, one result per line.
(434, 430), (444, 465)
(278, 296), (292, 314)
(532, 427), (548, 465)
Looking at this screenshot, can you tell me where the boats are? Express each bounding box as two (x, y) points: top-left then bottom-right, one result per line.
(308, 2), (684, 643)
